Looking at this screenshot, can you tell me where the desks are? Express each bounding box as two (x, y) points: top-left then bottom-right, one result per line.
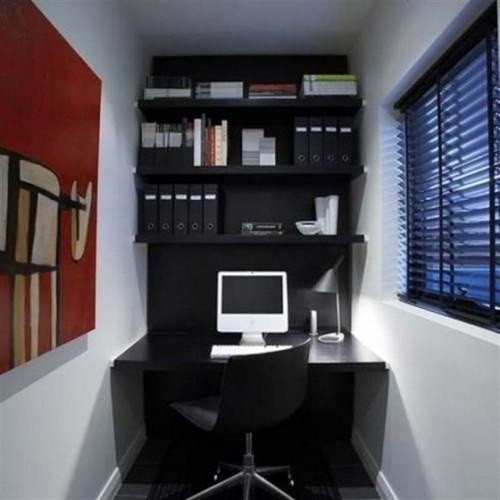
(114, 332), (387, 373)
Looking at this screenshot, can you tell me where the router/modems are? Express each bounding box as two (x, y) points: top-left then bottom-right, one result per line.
(309, 310), (319, 336)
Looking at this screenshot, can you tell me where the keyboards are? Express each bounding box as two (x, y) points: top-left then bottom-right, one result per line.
(210, 345), (293, 360)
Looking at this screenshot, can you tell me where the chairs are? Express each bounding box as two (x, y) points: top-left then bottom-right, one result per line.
(169, 337), (312, 500)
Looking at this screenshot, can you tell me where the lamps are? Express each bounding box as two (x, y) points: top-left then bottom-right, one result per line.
(313, 253), (345, 345)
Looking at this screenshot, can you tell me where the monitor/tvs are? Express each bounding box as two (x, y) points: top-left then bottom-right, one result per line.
(217, 271), (289, 345)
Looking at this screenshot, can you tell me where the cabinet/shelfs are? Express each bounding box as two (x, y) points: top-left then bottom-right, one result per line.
(133, 57), (366, 243)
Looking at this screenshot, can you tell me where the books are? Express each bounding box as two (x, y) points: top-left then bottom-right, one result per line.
(139, 73), (359, 166)
